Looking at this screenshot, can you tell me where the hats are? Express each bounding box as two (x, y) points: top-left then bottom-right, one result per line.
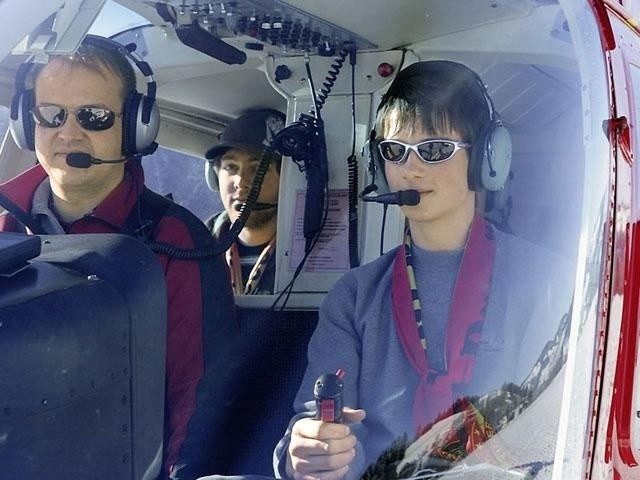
(205, 110), (285, 162)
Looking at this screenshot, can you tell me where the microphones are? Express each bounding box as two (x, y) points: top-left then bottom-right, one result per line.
(66, 141), (158, 168)
(234, 203), (277, 210)
(358, 185), (420, 205)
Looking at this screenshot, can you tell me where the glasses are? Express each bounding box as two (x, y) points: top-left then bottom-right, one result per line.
(377, 138), (474, 166)
(27, 102), (123, 132)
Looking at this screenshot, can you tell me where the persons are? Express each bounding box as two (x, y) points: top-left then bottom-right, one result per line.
(202, 108), (287, 297)
(274, 58), (578, 480)
(0, 34), (241, 480)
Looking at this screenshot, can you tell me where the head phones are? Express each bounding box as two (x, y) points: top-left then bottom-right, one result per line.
(10, 34), (160, 157)
(204, 108), (286, 193)
(359, 59), (512, 191)
